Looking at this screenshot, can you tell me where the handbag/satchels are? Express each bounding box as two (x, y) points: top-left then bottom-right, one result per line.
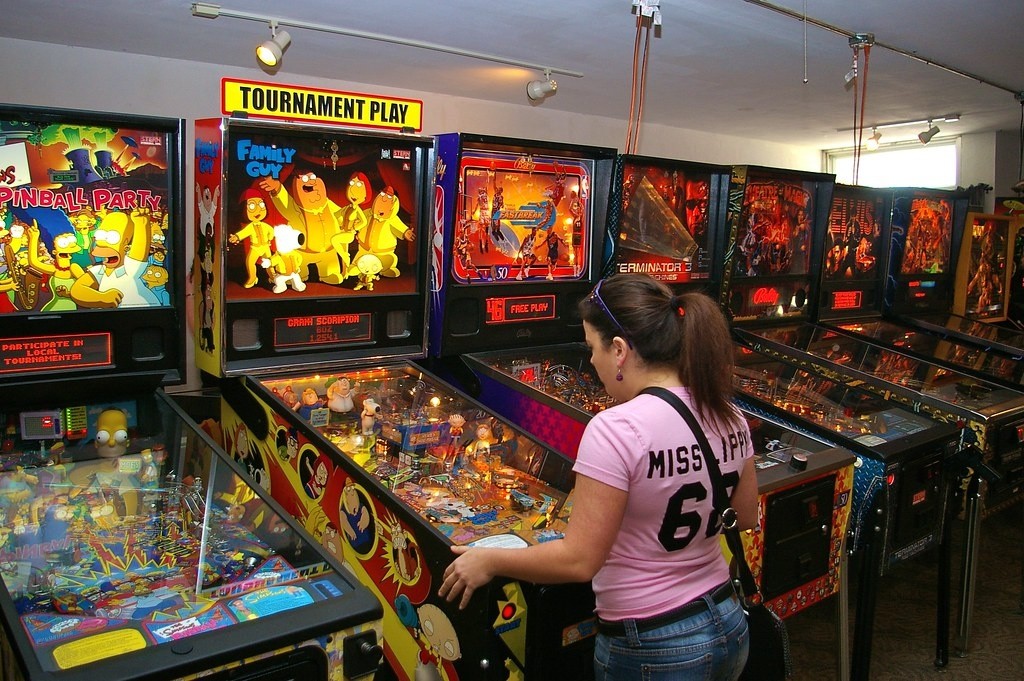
(734, 579), (791, 681)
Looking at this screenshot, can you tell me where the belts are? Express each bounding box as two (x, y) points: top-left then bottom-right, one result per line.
(597, 580), (734, 638)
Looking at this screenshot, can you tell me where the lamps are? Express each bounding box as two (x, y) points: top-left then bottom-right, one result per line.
(256, 21), (291, 66)
(867, 126), (882, 150)
(918, 120), (940, 145)
(527, 70), (556, 101)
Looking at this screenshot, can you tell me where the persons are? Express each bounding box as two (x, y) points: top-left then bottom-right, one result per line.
(326, 377), (360, 418)
(828, 207), (861, 279)
(471, 160), (584, 280)
(472, 424), (491, 458)
(439, 271), (758, 681)
(744, 203), (807, 273)
(447, 413), (465, 438)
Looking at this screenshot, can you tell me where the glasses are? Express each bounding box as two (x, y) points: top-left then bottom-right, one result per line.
(586, 280), (632, 355)
